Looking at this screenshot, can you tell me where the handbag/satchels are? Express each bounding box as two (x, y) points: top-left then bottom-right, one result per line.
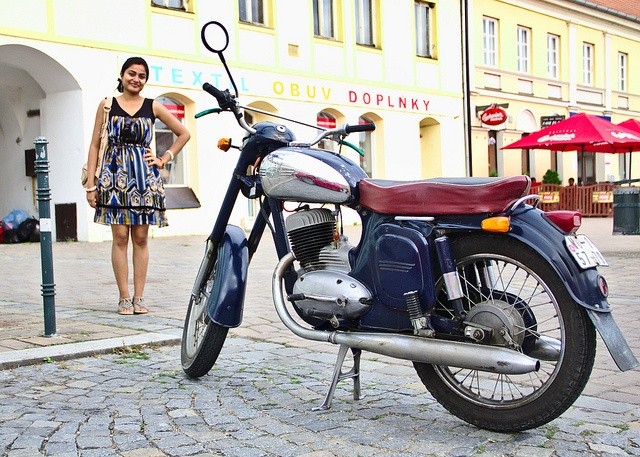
(81, 97), (113, 188)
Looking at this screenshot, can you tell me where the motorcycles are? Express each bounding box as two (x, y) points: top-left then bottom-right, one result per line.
(180, 21), (640, 433)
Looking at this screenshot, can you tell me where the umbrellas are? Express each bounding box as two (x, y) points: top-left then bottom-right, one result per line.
(578, 118), (640, 187)
(500, 112), (640, 187)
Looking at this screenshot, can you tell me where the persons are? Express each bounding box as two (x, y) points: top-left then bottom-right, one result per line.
(85, 57), (190, 315)
(569, 178), (574, 186)
(531, 178), (542, 187)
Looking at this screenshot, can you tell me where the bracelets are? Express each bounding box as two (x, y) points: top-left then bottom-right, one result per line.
(86, 186), (97, 192)
(158, 158), (164, 169)
(165, 150), (174, 160)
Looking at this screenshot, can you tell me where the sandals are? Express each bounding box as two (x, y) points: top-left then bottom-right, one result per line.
(118, 297), (134, 314)
(133, 296), (148, 313)
